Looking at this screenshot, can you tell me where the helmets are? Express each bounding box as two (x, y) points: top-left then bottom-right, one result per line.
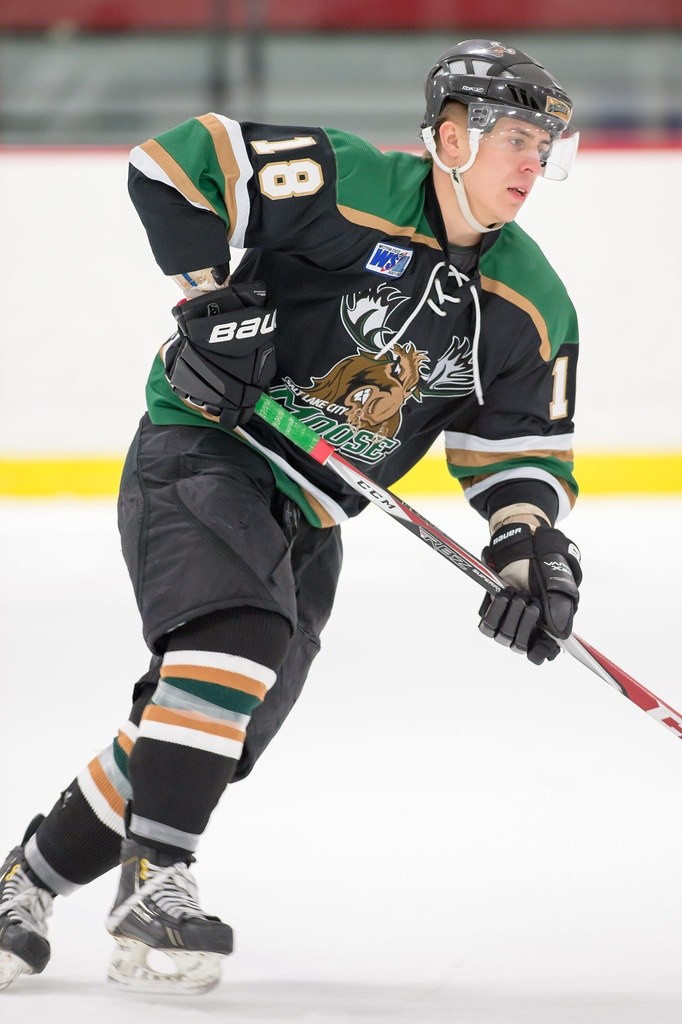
(420, 38), (573, 140)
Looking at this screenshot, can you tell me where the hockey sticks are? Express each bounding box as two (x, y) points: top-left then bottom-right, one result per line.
(253, 389), (682, 739)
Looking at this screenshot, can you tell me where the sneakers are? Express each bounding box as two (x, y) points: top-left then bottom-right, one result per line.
(105, 800), (233, 996)
(0, 813), (58, 990)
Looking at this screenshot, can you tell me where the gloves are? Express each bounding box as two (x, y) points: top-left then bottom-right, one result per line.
(164, 280), (277, 429)
(478, 522), (583, 665)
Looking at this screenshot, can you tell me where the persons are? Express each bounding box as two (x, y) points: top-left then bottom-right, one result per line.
(0, 40), (584, 996)
